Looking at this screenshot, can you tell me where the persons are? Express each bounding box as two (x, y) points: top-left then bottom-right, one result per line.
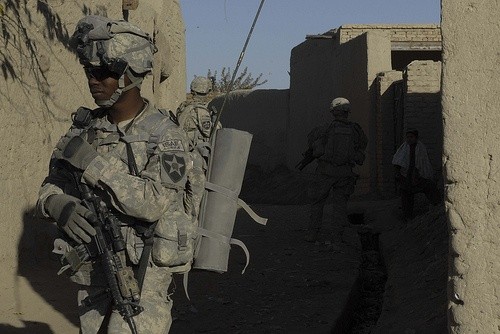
(177, 78), (222, 156)
(392, 127), (433, 223)
(302, 96), (370, 241)
(35, 15), (208, 334)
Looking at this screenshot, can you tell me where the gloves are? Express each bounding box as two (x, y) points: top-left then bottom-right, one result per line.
(44, 195), (97, 244)
(51, 135), (98, 168)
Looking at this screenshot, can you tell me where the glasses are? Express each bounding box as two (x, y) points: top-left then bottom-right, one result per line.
(81, 66), (111, 81)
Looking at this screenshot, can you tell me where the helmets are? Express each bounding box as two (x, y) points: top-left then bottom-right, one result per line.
(329, 97), (353, 111)
(77, 20), (156, 76)
(189, 76), (215, 93)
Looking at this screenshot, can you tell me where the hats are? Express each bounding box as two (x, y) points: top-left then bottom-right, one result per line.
(405, 127), (418, 132)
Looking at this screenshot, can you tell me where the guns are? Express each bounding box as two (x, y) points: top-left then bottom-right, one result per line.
(58, 163), (147, 334)
(294, 145), (320, 171)
(210, 106), (219, 123)
